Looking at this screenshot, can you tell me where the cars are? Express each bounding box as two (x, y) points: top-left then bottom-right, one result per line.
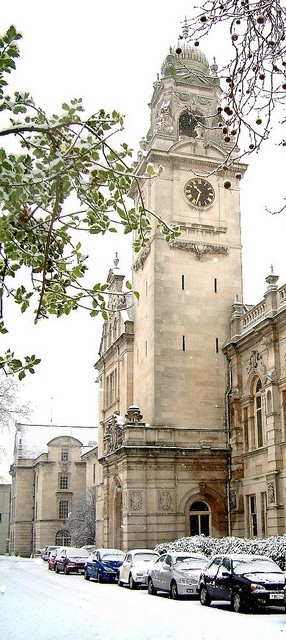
(145, 551), (210, 599)
(47, 548), (62, 570)
(53, 547), (90, 575)
(198, 553), (286, 613)
(117, 549), (161, 590)
(83, 548), (126, 583)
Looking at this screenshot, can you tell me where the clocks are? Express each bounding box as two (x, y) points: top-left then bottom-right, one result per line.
(185, 176), (215, 209)
